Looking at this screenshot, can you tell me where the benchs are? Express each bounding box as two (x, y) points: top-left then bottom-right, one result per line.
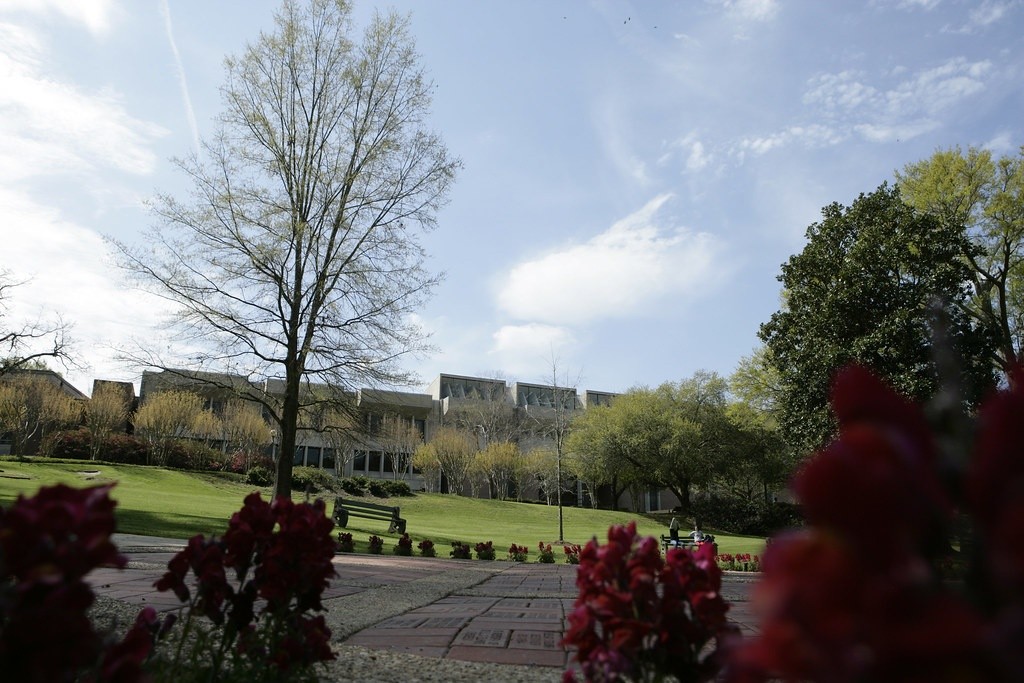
(659, 533), (718, 555)
(668, 506), (682, 514)
(332, 496), (407, 536)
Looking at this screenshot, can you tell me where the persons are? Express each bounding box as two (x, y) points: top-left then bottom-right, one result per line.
(688, 525), (704, 546)
(669, 517), (686, 550)
